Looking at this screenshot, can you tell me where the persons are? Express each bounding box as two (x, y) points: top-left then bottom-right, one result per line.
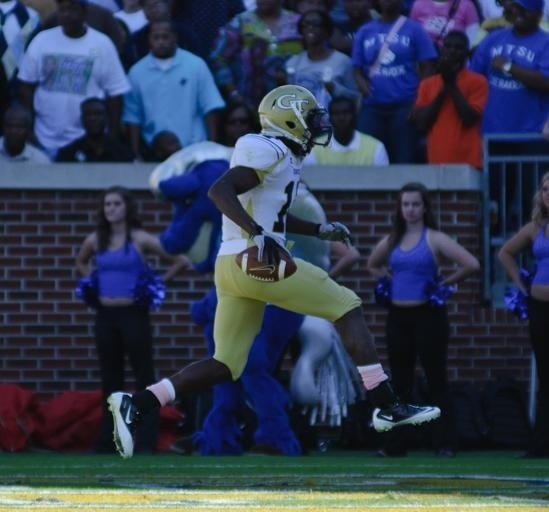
(367, 182), (480, 458)
(106, 84), (441, 461)
(327, 237), (360, 282)
(75, 184), (189, 457)
(498, 172), (549, 456)
(2, 2), (547, 241)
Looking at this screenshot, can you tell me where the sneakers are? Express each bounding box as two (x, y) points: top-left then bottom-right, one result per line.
(377, 439), (457, 458)
(107, 390), (139, 460)
(369, 403), (442, 433)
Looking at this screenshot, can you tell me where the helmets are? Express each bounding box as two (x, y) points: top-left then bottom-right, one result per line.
(257, 84), (325, 154)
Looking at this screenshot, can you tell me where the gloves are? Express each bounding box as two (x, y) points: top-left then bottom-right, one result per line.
(248, 227), (293, 266)
(319, 220), (353, 250)
